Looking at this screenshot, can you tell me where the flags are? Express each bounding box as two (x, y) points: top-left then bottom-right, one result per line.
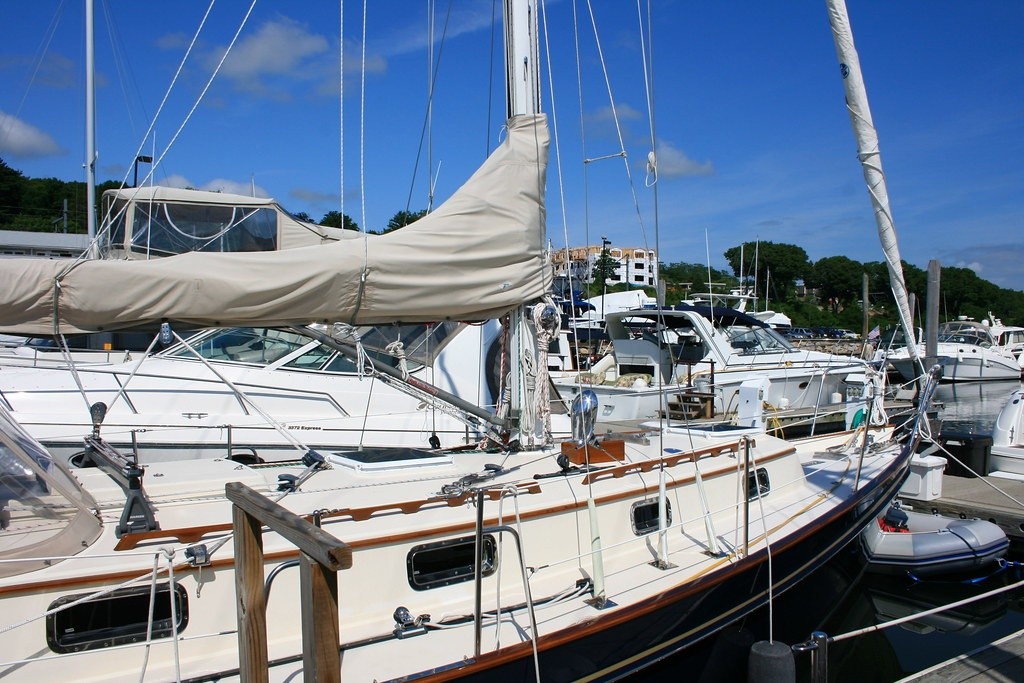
(868, 326), (880, 340)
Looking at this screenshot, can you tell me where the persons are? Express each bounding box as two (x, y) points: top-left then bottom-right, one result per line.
(865, 341), (877, 362)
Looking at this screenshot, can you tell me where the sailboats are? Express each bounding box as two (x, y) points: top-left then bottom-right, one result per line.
(0, 0), (1024, 683)
